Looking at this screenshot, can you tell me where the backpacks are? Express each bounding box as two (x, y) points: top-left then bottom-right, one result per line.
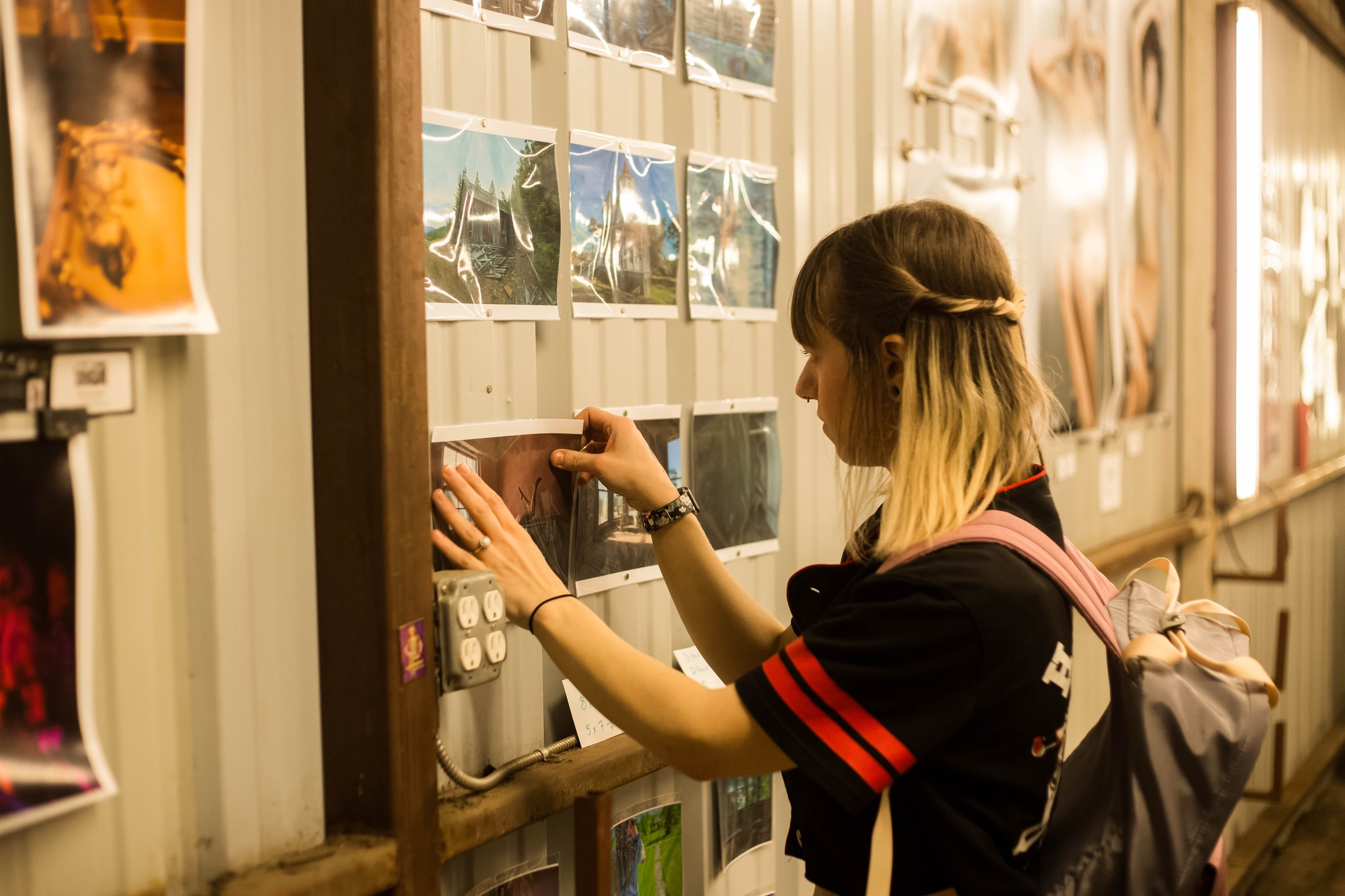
(875, 514), (1281, 896)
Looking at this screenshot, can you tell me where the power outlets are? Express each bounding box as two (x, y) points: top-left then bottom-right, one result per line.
(433, 571), (508, 697)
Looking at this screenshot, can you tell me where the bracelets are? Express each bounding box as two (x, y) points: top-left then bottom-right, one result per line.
(529, 594), (577, 635)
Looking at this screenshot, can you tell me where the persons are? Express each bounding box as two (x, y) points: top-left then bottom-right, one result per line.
(912, 0), (1169, 431)
(433, 200), (1072, 896)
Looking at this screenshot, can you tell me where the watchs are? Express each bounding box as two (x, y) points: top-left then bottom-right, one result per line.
(639, 487), (700, 535)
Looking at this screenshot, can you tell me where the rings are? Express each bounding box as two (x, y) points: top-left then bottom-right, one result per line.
(473, 535), (490, 554)
